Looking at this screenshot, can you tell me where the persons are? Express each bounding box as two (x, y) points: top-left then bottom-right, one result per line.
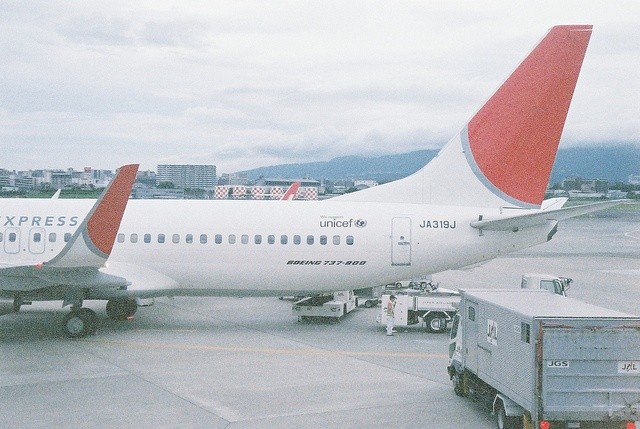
(385, 293), (398, 337)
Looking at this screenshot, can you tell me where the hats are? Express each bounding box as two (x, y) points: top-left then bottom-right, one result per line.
(390, 295), (396, 300)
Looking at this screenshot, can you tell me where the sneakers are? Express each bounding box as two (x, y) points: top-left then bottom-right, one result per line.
(387, 334), (394, 336)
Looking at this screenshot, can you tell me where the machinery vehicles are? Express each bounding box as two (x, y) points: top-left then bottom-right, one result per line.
(292, 290), (358, 324)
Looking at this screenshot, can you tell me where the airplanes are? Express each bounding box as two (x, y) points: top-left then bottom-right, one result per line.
(0, 25), (639, 337)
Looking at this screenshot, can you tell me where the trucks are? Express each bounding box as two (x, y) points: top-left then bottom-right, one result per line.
(375, 274), (573, 333)
(447, 288), (640, 429)
